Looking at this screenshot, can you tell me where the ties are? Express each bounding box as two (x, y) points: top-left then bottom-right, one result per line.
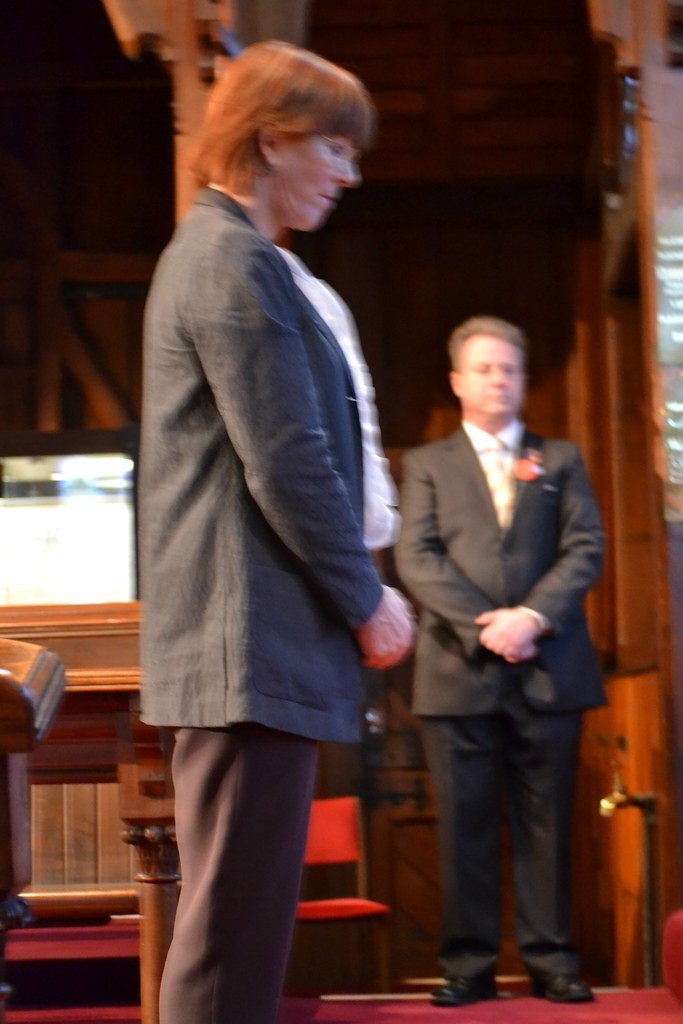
(487, 445), (517, 534)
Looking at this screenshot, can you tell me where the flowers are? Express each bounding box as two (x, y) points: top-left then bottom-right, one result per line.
(515, 446), (550, 483)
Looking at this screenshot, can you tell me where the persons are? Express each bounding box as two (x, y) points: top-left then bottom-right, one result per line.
(393, 314), (606, 1008)
(140, 40), (415, 1024)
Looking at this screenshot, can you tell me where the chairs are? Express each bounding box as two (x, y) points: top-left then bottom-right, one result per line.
(285, 795), (393, 996)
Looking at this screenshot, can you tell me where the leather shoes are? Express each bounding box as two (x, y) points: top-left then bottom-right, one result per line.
(431, 972), (498, 1006)
(529, 976), (591, 1002)
